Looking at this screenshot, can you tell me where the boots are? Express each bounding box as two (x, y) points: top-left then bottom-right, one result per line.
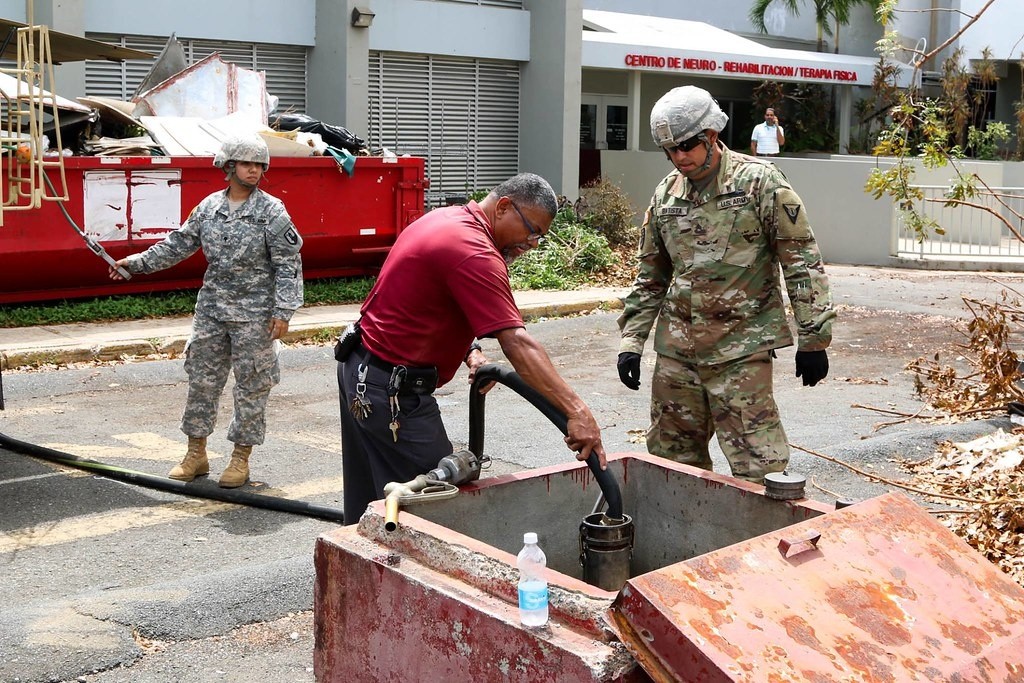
(168, 436), (209, 481)
(218, 443), (252, 487)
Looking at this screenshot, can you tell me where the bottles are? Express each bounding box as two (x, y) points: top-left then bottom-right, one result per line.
(516, 532), (548, 627)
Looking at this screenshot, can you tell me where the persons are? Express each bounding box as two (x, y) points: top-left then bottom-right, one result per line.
(616, 86), (832, 485)
(336, 172), (609, 538)
(751, 108), (785, 156)
(111, 132), (303, 487)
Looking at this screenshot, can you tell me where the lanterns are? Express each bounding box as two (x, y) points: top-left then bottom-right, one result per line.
(349, 391), (373, 419)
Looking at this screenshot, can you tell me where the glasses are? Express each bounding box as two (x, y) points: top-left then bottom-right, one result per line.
(666, 129), (707, 152)
(511, 201), (547, 243)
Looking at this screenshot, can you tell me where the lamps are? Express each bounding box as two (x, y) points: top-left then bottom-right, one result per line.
(352, 6), (376, 28)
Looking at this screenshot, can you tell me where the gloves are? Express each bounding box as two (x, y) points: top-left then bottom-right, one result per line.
(617, 352), (641, 390)
(795, 351), (829, 387)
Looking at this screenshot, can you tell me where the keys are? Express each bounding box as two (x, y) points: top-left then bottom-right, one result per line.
(387, 387), (407, 442)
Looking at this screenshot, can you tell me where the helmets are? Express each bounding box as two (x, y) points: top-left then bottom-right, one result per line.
(650, 85), (730, 148)
(213, 132), (270, 172)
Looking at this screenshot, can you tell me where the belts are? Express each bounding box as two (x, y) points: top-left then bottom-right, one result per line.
(354, 343), (436, 381)
